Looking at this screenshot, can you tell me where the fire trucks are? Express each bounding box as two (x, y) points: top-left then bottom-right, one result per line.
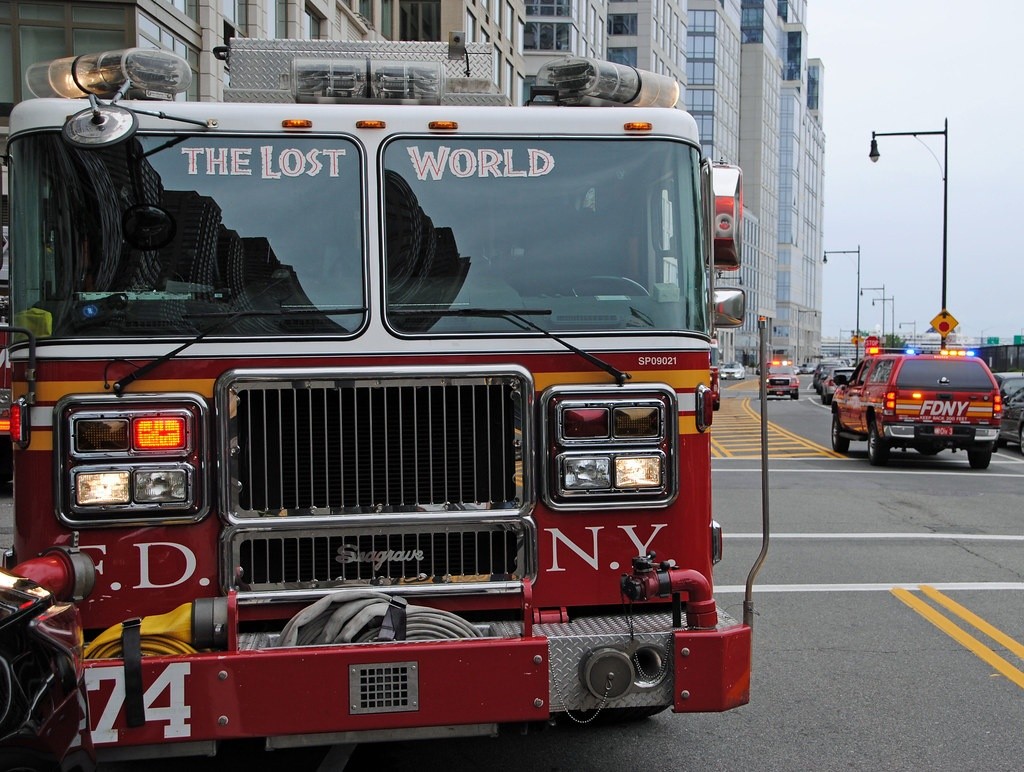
(0, 35), (781, 772)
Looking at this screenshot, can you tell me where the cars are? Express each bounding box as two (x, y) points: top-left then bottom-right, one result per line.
(720, 362), (745, 380)
(992, 386), (1024, 455)
(793, 365), (801, 376)
(799, 362), (819, 374)
(811, 357), (862, 405)
(991, 372), (1024, 397)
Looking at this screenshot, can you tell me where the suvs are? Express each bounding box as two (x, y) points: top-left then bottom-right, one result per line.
(759, 360), (799, 400)
(829, 348), (1003, 469)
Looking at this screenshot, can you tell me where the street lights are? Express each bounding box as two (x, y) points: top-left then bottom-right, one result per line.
(860, 284), (886, 349)
(823, 245), (861, 366)
(869, 117), (949, 351)
(898, 320), (917, 348)
(871, 295), (895, 348)
